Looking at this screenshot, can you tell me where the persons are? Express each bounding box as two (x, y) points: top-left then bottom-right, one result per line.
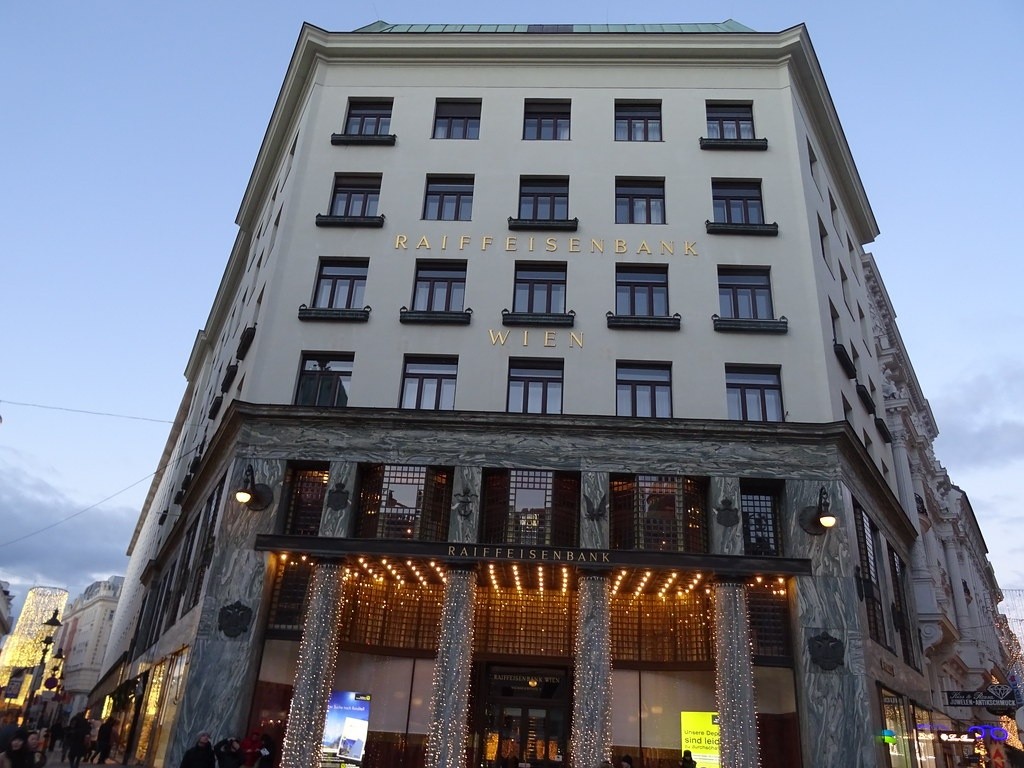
(679, 750), (697, 768)
(620, 755), (632, 768)
(0, 710), (121, 768)
(180, 730), (276, 767)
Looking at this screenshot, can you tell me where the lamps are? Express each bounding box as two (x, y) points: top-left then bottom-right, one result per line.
(236, 463), (273, 510)
(799, 486), (836, 536)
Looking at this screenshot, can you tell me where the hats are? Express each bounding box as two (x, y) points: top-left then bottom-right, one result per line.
(197, 731), (210, 740)
(623, 755), (632, 765)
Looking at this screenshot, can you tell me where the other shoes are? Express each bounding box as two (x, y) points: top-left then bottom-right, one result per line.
(90, 759), (93, 763)
(97, 761), (105, 764)
(83, 758), (88, 762)
(61, 755), (65, 762)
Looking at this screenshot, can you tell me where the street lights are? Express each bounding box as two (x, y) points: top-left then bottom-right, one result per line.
(36, 647), (66, 731)
(20, 608), (67, 730)
